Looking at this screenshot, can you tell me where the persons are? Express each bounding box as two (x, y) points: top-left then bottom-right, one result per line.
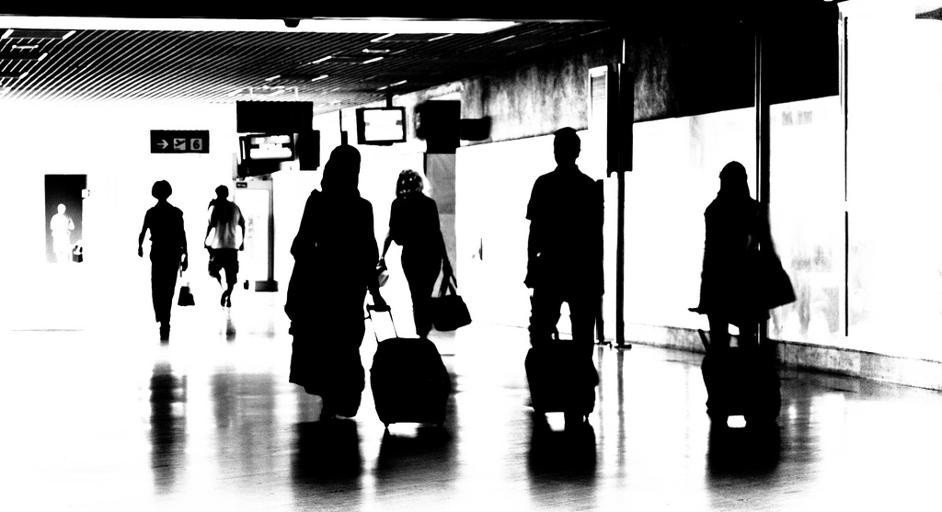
(522, 126), (602, 389)
(138, 180), (188, 343)
(50, 198), (76, 262)
(380, 167), (447, 342)
(696, 160), (776, 345)
(284, 143), (385, 421)
(202, 184), (246, 308)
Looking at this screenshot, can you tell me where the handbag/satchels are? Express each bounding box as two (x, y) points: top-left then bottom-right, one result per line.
(431, 294), (472, 331)
(178, 286), (195, 306)
(759, 250), (795, 308)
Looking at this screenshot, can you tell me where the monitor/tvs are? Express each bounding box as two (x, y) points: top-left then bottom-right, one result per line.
(356, 107), (408, 144)
(588, 66), (614, 179)
(245, 132), (298, 161)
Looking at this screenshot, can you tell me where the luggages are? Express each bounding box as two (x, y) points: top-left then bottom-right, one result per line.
(525, 296), (599, 417)
(365, 304), (451, 427)
(689, 308), (781, 422)
